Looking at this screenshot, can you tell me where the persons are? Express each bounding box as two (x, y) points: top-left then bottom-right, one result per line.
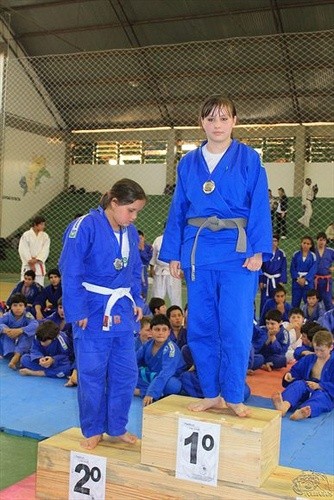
(133, 178), (334, 421)
(58, 178), (149, 450)
(0, 213), (84, 387)
(161, 94), (273, 419)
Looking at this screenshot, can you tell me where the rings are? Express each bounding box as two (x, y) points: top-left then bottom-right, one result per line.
(144, 401), (146, 403)
